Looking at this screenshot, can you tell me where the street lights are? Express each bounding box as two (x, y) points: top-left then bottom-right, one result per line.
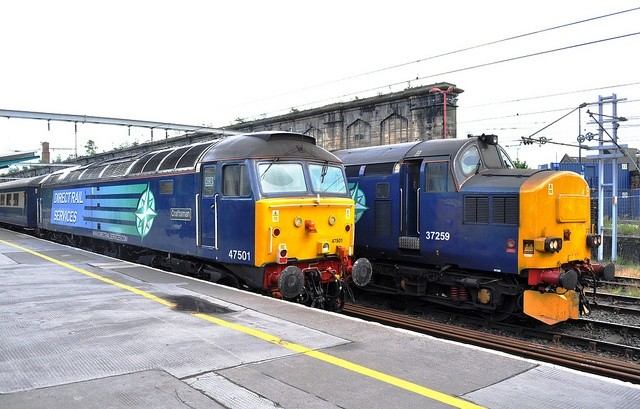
(429, 86), (457, 139)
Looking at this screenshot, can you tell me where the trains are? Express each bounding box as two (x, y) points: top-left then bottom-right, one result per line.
(326, 134), (615, 327)
(0, 130), (357, 313)
